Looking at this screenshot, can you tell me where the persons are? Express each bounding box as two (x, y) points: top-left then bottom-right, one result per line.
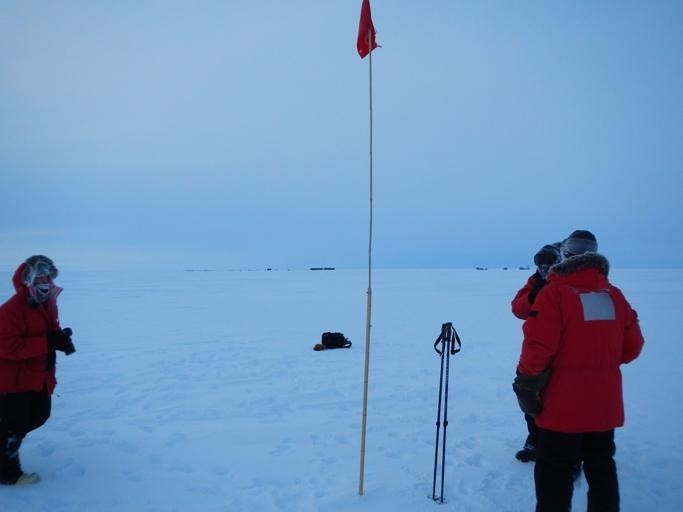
(511, 228), (645, 512)
(0, 254), (76, 487)
(509, 239), (565, 466)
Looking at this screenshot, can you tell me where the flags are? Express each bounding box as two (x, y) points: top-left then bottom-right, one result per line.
(355, 0), (382, 59)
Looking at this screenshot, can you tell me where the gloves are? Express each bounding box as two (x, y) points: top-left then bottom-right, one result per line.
(512, 372), (548, 415)
(49, 328), (76, 355)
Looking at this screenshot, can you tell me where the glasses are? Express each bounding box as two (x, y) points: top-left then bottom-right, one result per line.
(533, 254), (554, 264)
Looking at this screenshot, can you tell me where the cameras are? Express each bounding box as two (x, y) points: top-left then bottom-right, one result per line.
(54, 327), (76, 356)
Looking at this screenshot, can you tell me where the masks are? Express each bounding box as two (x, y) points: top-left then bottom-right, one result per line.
(33, 284), (49, 302)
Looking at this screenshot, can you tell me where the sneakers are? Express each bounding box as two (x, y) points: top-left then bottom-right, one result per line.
(515, 449), (537, 461)
(16, 472), (40, 484)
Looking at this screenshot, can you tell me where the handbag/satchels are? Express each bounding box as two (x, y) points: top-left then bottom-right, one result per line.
(321, 332), (352, 349)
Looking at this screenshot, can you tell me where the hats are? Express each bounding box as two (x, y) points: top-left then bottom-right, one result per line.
(24, 255), (58, 286)
(538, 229), (597, 259)
